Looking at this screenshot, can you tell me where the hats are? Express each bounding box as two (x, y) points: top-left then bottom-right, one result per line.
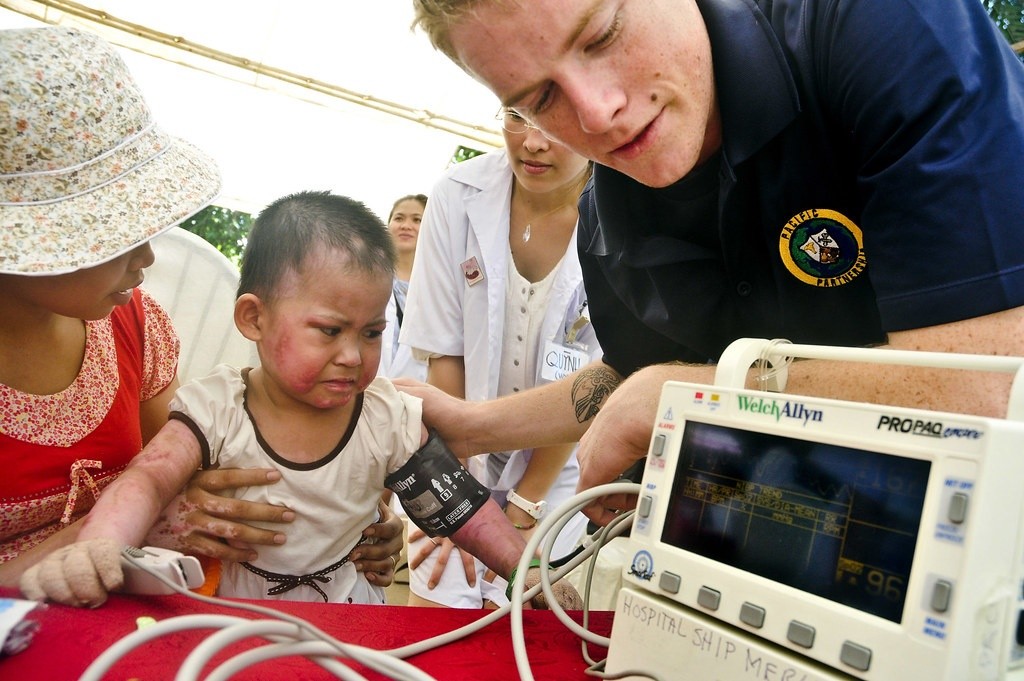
(0, 26), (223, 277)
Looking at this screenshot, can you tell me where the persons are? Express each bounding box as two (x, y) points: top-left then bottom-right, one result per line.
(0, 24), (405, 593)
(377, 0), (1023, 609)
(17, 188), (584, 609)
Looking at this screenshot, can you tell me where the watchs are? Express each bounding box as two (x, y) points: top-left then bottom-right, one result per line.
(504, 488), (549, 520)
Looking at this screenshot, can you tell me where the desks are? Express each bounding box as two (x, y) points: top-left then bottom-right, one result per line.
(0, 585), (615, 681)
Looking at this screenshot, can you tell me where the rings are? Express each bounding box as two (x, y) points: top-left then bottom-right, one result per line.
(389, 555), (396, 566)
(429, 537), (438, 546)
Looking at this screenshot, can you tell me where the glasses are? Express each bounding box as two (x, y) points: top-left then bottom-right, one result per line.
(495, 106), (539, 134)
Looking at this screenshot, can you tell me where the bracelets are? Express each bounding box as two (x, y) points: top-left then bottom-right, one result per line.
(502, 507), (538, 530)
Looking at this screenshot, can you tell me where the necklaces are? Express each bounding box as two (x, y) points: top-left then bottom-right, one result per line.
(515, 171), (593, 244)
